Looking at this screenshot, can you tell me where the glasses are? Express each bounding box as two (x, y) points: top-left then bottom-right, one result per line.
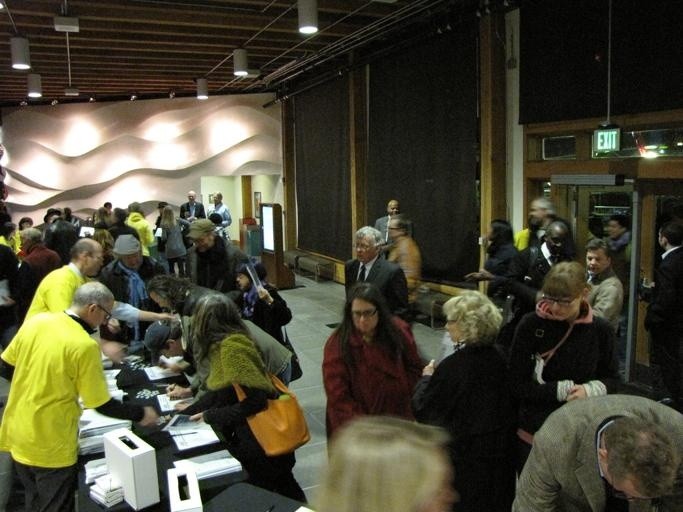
(89, 303), (112, 321)
(541, 293), (577, 309)
(351, 308), (377, 320)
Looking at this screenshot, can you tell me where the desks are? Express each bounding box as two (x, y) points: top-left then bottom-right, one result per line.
(77, 349), (317, 512)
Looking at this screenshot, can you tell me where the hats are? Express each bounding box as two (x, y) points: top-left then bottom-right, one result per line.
(144, 319), (171, 363)
(186, 218), (216, 240)
(111, 234), (141, 256)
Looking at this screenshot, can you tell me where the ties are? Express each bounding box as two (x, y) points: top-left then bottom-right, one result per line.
(358, 265), (366, 282)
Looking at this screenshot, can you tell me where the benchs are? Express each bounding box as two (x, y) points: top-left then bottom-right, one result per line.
(410, 287), (456, 330)
(284, 250), (310, 273)
(297, 256), (336, 282)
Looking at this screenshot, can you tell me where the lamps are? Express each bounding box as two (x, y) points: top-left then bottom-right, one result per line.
(27, 74), (42, 99)
(297, 0), (319, 34)
(233, 49), (249, 76)
(10, 37), (31, 70)
(196, 78), (209, 100)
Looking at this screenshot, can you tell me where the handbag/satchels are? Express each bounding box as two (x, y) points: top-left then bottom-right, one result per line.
(283, 339), (303, 382)
(246, 392), (311, 458)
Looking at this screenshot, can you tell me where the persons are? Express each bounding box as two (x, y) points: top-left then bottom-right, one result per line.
(322, 192), (682, 512)
(2, 189), (308, 512)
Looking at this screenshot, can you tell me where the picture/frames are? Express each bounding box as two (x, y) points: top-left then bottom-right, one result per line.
(254, 192), (262, 218)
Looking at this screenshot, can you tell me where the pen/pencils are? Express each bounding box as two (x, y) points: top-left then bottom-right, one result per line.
(172, 379), (179, 391)
